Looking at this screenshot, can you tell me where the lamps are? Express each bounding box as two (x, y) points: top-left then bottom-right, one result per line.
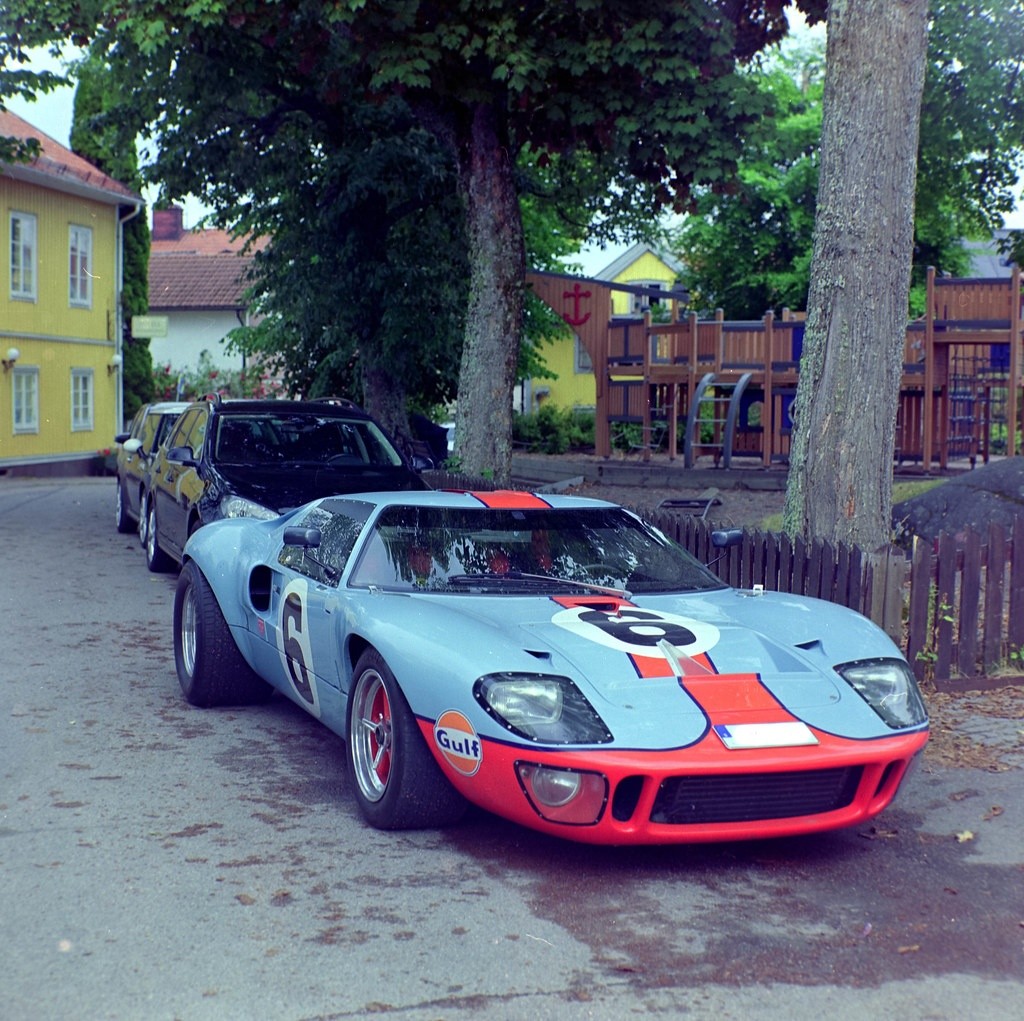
(107, 354), (122, 377)
(2, 347), (20, 370)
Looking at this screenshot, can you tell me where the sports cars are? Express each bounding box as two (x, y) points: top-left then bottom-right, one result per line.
(169, 483), (933, 851)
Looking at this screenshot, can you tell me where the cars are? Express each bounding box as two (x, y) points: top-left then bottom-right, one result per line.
(135, 390), (444, 575)
(112, 399), (287, 532)
